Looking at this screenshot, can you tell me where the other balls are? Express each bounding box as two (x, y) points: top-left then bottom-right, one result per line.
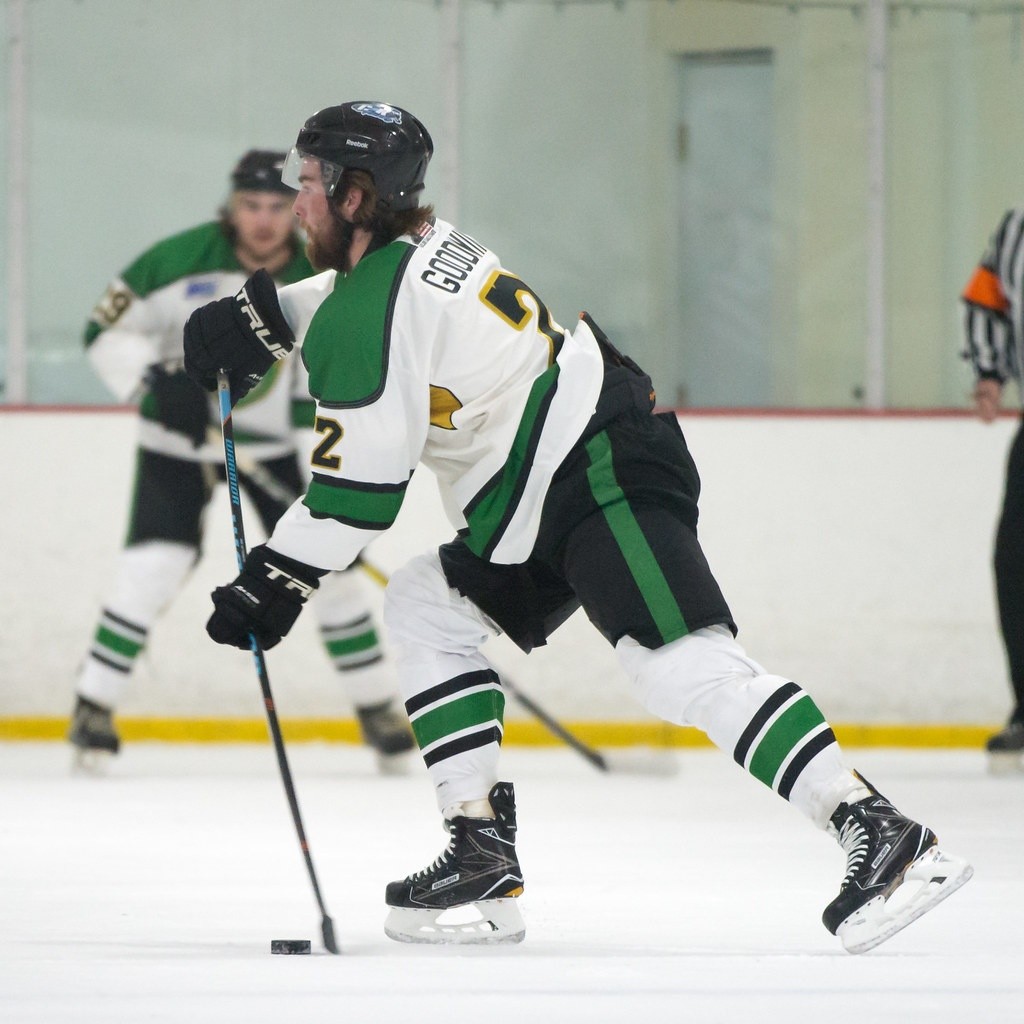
(269, 938), (313, 956)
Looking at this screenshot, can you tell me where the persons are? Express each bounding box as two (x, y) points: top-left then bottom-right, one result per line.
(206, 99), (972, 951)
(64, 149), (420, 777)
(959, 200), (1024, 755)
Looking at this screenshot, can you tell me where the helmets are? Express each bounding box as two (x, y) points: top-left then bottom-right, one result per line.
(234, 150), (299, 196)
(281, 101), (433, 210)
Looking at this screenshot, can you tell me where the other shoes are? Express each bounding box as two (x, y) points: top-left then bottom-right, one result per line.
(360, 701), (417, 771)
(69, 698), (119, 768)
(988, 720), (1024, 754)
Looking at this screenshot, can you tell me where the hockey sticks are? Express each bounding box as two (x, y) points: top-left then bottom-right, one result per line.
(215, 364), (366, 956)
(142, 361), (693, 779)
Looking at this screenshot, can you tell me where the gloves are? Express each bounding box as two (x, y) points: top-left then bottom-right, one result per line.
(205, 545), (329, 651)
(183, 267), (296, 411)
(144, 363), (211, 446)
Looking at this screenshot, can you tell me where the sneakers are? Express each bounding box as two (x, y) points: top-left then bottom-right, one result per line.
(823, 767), (974, 956)
(385, 782), (526, 945)
